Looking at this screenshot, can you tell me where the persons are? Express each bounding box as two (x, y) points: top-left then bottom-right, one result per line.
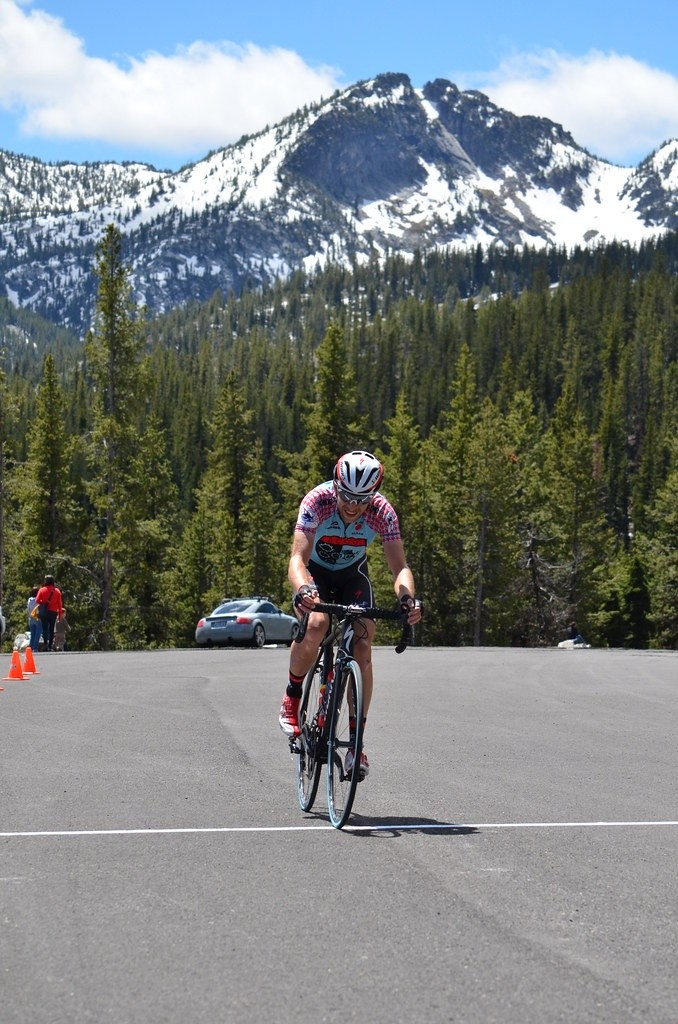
(26, 587), (43, 652)
(36, 574), (62, 650)
(54, 608), (71, 652)
(278, 451), (423, 776)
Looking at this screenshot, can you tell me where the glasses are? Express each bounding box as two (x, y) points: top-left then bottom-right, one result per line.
(338, 488), (374, 504)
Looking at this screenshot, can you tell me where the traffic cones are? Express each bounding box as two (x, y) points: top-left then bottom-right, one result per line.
(22, 647), (40, 675)
(3, 651), (29, 680)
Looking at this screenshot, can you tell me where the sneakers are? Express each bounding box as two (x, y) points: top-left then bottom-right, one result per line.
(343, 749), (369, 776)
(277, 691), (301, 736)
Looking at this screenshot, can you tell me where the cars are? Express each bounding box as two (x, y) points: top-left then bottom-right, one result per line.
(196, 595), (301, 647)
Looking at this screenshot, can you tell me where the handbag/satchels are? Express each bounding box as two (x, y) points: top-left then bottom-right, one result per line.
(36, 602), (48, 617)
(31, 603), (40, 621)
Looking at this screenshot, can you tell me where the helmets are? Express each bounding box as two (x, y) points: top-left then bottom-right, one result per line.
(333, 451), (385, 495)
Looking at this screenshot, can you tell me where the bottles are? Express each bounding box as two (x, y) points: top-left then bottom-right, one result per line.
(318, 685), (327, 728)
(323, 678), (334, 720)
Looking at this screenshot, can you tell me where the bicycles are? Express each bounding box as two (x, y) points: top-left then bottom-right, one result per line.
(288, 590), (416, 830)
